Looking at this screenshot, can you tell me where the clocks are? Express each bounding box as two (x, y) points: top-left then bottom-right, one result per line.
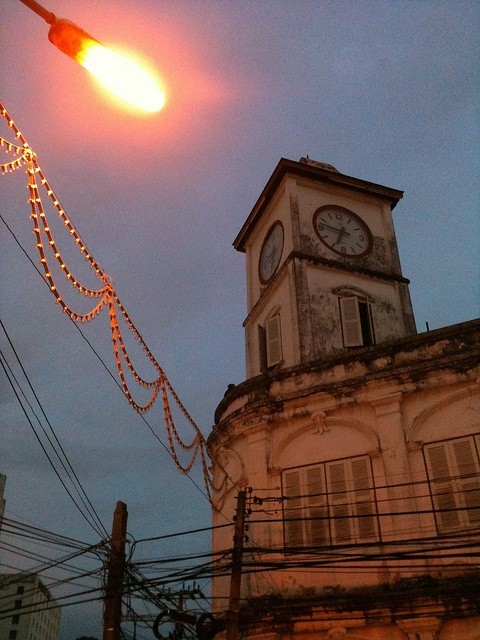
(254, 219), (286, 286)
(310, 204), (374, 260)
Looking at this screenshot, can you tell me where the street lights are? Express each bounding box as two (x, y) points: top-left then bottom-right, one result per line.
(18, 0), (169, 118)
